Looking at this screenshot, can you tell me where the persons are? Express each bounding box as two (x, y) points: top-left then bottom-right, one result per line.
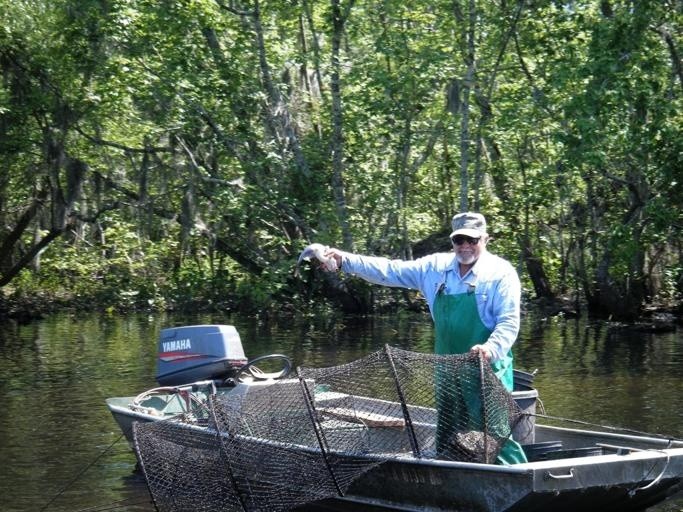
(319, 210), (531, 467)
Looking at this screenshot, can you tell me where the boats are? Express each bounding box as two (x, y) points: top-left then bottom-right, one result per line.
(104, 323), (683, 512)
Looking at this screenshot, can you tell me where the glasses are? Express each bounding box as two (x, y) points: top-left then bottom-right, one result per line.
(452, 235), (479, 244)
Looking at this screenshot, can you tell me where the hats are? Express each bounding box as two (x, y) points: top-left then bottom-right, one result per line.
(449, 212), (486, 238)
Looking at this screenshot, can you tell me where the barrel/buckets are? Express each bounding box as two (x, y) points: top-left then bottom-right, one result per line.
(511, 369), (603, 460)
